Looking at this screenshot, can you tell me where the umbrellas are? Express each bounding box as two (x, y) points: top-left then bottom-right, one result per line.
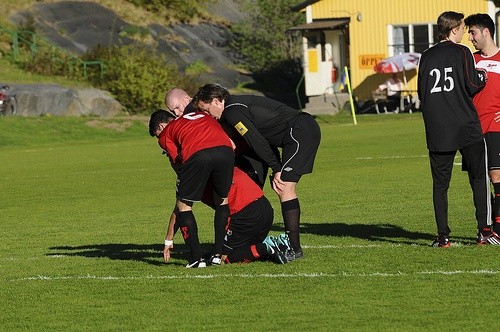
(373, 51), (425, 114)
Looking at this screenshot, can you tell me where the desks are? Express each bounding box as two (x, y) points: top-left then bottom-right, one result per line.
(395, 89), (420, 114)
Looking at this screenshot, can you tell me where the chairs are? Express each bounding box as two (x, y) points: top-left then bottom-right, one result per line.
(372, 88), (391, 115)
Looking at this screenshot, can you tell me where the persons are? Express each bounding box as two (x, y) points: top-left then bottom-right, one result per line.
(375, 73), (405, 114)
(463, 12), (500, 239)
(149, 109), (237, 270)
(161, 149), (296, 264)
(164, 87), (274, 262)
(0, 83), (11, 108)
(417, 10), (500, 248)
(189, 82), (321, 261)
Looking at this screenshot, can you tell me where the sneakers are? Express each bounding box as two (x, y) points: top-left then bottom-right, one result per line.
(475, 234), (490, 244)
(277, 234), (296, 262)
(262, 235), (287, 264)
(487, 233), (500, 246)
(432, 240), (451, 248)
(186, 253), (222, 268)
